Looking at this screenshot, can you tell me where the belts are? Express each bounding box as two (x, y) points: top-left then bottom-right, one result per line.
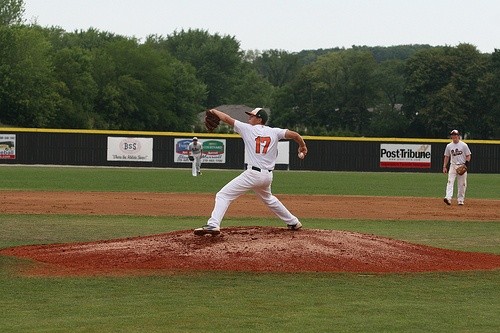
(252, 166), (272, 172)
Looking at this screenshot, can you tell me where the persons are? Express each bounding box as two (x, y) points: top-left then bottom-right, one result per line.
(443, 130), (471, 206)
(188, 137), (203, 176)
(194, 107), (308, 235)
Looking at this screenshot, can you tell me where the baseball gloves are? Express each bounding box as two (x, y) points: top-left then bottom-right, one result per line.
(189, 156), (194, 161)
(205, 110), (220, 131)
(456, 165), (468, 176)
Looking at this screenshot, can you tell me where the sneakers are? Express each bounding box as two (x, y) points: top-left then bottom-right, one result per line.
(194, 225), (221, 236)
(287, 221), (302, 230)
(458, 200), (464, 204)
(444, 198), (451, 205)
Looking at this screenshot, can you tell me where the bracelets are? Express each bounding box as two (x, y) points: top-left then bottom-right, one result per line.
(443, 167), (447, 168)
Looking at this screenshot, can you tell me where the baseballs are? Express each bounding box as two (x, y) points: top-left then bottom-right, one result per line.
(298, 152), (304, 159)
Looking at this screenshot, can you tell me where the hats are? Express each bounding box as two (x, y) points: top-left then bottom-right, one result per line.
(245, 108), (268, 122)
(451, 130), (459, 135)
(193, 136), (198, 141)
